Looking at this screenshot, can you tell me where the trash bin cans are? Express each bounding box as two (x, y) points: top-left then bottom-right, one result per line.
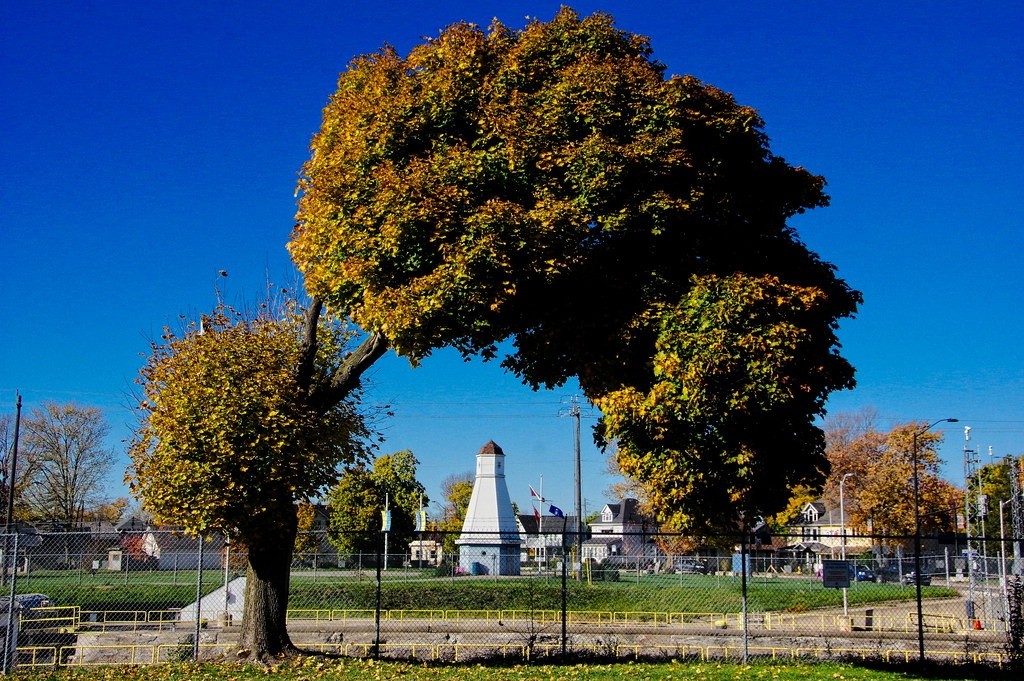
(865, 608), (883, 631)
(472, 562), (479, 575)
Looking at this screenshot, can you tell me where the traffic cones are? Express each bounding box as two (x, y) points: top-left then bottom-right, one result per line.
(973, 617), (983, 629)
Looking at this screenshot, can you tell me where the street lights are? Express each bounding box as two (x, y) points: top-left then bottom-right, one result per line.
(914, 418), (959, 661)
(839, 472), (853, 616)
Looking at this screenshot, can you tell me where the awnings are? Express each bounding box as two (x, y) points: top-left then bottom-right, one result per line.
(815, 546), (869, 555)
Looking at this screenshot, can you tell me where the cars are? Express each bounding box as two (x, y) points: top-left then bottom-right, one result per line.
(876, 562), (930, 587)
(0, 593), (77, 669)
(850, 564), (877, 583)
(673, 559), (709, 575)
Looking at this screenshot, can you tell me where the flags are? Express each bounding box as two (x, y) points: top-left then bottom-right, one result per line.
(531, 486), (540, 496)
(534, 506), (538, 528)
(549, 505), (564, 519)
